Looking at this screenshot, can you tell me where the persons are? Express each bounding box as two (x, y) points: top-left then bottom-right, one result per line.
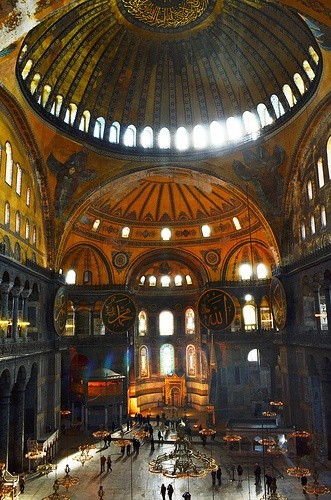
(135, 414), (163, 450)
(104, 434), (111, 447)
(98, 486), (104, 500)
(301, 475), (308, 494)
(107, 457), (112, 473)
(166, 416), (174, 428)
(127, 444), (131, 455)
(160, 484), (173, 500)
(182, 492), (191, 500)
(211, 467), (222, 486)
(133, 438), (140, 454)
(265, 473), (277, 495)
(176, 415), (192, 441)
(126, 418), (130, 431)
(255, 466), (261, 485)
(20, 476), (25, 494)
(121, 445), (125, 456)
(198, 425), (216, 447)
(237, 465), (243, 483)
(101, 455), (106, 471)
